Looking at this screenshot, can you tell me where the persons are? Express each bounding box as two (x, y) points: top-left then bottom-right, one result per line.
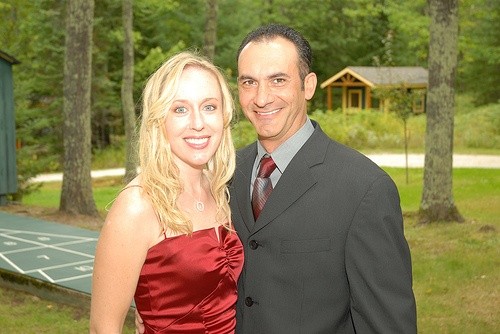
(90, 51), (248, 334)
(132, 23), (418, 334)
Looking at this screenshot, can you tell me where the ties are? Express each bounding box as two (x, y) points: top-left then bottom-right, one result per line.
(251, 156), (277, 222)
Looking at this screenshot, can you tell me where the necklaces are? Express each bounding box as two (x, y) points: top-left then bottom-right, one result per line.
(182, 173), (204, 211)
